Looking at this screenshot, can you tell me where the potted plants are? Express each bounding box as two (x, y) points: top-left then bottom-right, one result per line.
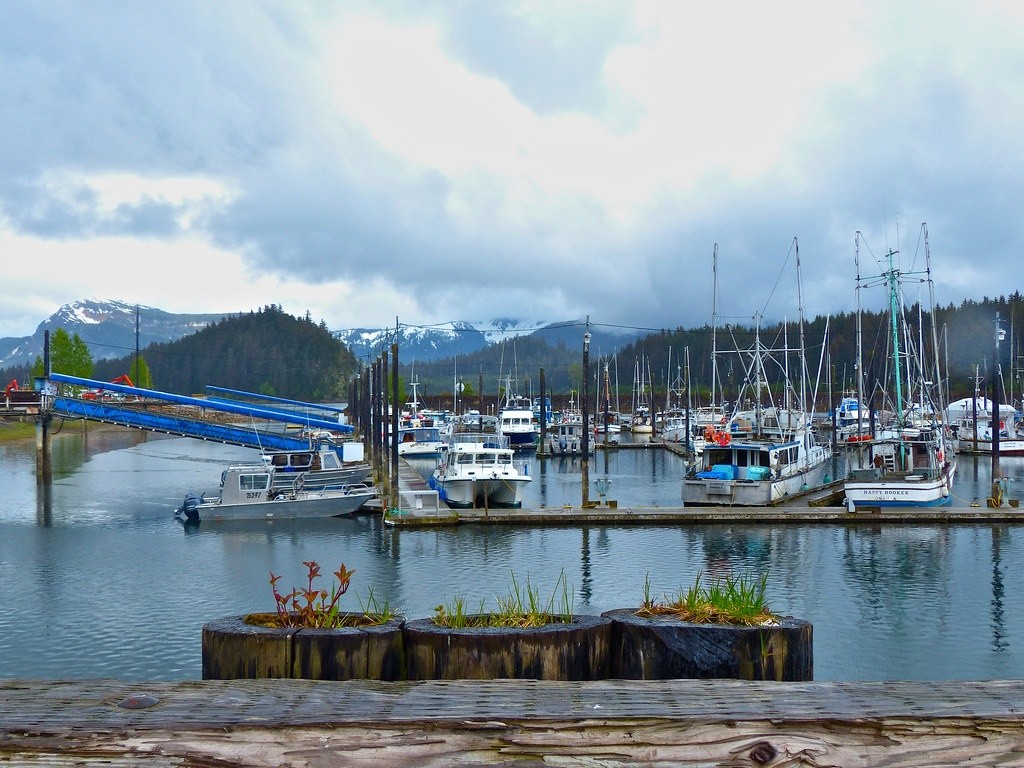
(400, 568), (612, 684)
(200, 560), (409, 684)
(599, 567), (815, 682)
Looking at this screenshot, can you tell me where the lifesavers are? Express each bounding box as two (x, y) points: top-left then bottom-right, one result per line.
(998, 421), (1005, 430)
(404, 414), (424, 420)
(923, 473), (929, 479)
(716, 432), (730, 446)
(848, 435), (870, 441)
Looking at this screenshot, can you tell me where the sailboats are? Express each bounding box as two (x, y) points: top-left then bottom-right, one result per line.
(171, 219), (1023, 526)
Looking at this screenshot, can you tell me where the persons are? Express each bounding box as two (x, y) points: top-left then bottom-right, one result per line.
(874, 454), (882, 468)
